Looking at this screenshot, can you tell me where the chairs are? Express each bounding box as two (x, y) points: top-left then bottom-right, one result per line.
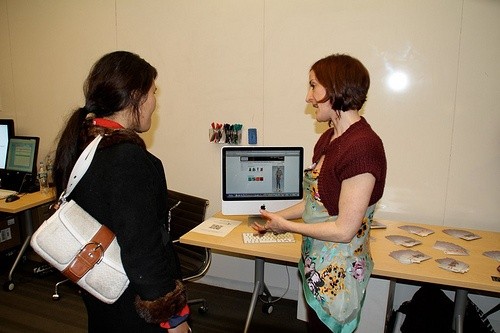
(167, 190), (212, 315)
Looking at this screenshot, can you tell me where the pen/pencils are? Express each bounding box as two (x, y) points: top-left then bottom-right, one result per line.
(225, 123), (243, 144)
(209, 122), (225, 143)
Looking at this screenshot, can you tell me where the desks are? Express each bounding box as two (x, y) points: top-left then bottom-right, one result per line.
(180, 210), (499, 333)
(0, 185), (57, 292)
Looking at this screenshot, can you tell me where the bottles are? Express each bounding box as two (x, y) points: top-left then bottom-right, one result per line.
(38, 162), (49, 193)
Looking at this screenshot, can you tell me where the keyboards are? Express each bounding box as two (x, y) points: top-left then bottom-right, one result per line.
(242, 233), (296, 244)
(0, 188), (17, 199)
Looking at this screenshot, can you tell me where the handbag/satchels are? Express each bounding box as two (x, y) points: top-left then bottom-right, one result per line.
(30, 134), (130, 305)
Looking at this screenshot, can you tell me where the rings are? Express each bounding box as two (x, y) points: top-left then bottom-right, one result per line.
(273, 232), (278, 235)
(188, 327), (192, 333)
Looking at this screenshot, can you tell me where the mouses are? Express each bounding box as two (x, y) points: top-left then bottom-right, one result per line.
(6, 194), (20, 203)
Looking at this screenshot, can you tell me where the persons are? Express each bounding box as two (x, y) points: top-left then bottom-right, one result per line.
(251, 52), (387, 333)
(275, 166), (284, 192)
(47, 50), (195, 333)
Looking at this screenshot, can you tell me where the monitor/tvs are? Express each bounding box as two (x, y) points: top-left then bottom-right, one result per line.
(0, 119), (15, 178)
(221, 146), (304, 228)
